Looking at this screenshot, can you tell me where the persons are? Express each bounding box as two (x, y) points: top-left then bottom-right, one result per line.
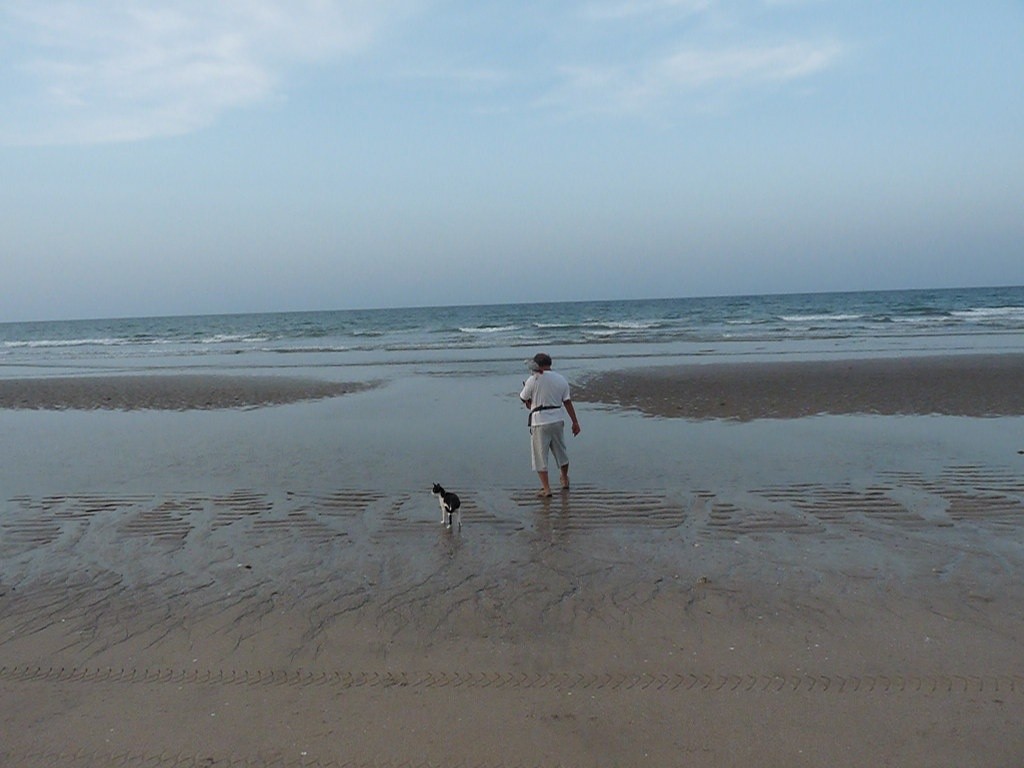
(520, 353), (580, 497)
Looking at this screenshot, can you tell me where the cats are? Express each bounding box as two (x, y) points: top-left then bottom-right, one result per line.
(431, 483), (462, 529)
